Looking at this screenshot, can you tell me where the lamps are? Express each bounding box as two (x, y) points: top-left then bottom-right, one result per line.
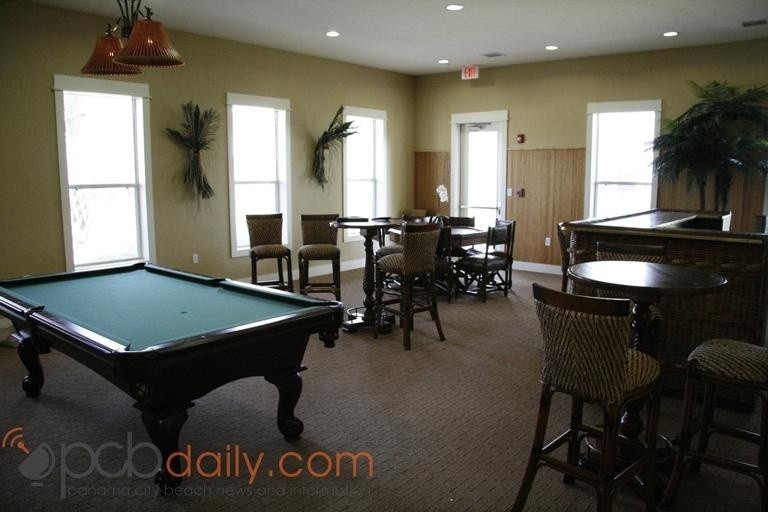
(80, 0), (185, 77)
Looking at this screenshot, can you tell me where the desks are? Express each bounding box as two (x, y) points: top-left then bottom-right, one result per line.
(566, 260), (728, 457)
(0, 260), (344, 499)
(341, 219), (407, 333)
(388, 227), (489, 296)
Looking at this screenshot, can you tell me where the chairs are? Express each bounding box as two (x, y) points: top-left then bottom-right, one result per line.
(556, 222), (571, 291)
(246, 215), (294, 293)
(374, 223), (446, 349)
(297, 215), (339, 303)
(511, 282), (670, 511)
(661, 338), (767, 512)
(471, 219), (516, 290)
(455, 226), (510, 304)
(446, 217), (474, 284)
